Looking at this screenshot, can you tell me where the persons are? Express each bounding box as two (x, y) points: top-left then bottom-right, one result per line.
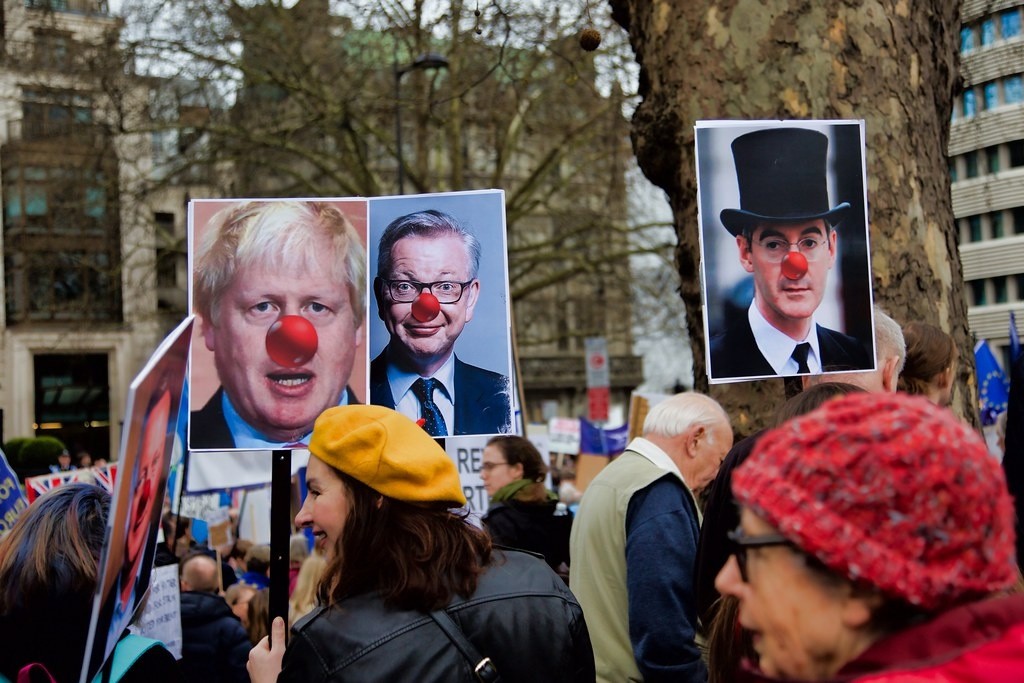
(87, 366), (170, 683)
(369, 210), (512, 436)
(707, 127), (875, 378)
(0, 307), (1023, 683)
(190, 199), (369, 450)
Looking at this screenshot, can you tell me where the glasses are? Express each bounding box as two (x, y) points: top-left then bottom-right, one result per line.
(729, 531), (794, 583)
(750, 237), (826, 256)
(485, 462), (510, 470)
(379, 276), (475, 303)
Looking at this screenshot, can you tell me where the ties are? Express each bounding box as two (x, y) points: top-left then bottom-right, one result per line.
(284, 442), (306, 447)
(410, 377), (446, 436)
(791, 342), (810, 373)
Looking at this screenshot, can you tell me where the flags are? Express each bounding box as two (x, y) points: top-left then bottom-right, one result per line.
(973, 338), (1011, 427)
(1008, 312), (1021, 365)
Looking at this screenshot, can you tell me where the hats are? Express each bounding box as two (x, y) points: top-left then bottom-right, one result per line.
(309, 403), (466, 507)
(729, 392), (1019, 608)
(719, 128), (850, 237)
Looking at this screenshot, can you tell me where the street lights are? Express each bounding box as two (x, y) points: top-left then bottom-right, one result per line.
(391, 53), (451, 197)
